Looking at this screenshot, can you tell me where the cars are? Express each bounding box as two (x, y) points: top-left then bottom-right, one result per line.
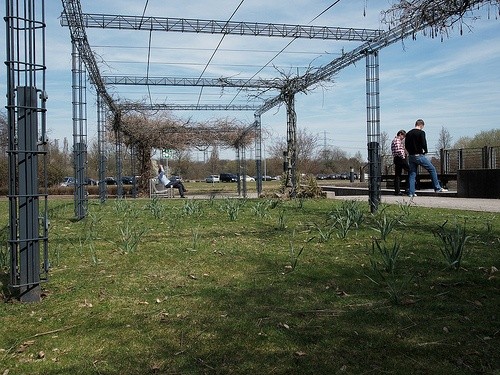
(60, 177), (78, 188)
(85, 178), (97, 185)
(317, 173), (358, 180)
(121, 176), (137, 184)
(172, 176), (184, 183)
(240, 175), (256, 182)
(98, 177), (119, 185)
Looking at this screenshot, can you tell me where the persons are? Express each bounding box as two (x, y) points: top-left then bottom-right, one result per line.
(158, 165), (188, 198)
(391, 130), (410, 196)
(405, 119), (448, 197)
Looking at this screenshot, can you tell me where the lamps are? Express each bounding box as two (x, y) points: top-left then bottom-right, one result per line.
(350, 168), (354, 173)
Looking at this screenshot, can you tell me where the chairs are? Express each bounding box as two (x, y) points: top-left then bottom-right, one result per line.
(149, 178), (174, 199)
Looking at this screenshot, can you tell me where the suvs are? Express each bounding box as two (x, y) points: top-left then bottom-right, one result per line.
(220, 173), (239, 182)
(206, 175), (219, 183)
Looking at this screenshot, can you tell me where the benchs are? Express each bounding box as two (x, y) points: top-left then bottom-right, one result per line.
(379, 175), (457, 189)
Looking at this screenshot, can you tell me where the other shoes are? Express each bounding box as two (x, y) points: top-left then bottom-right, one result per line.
(436, 188), (449, 193)
(409, 193), (417, 197)
(396, 193), (405, 196)
